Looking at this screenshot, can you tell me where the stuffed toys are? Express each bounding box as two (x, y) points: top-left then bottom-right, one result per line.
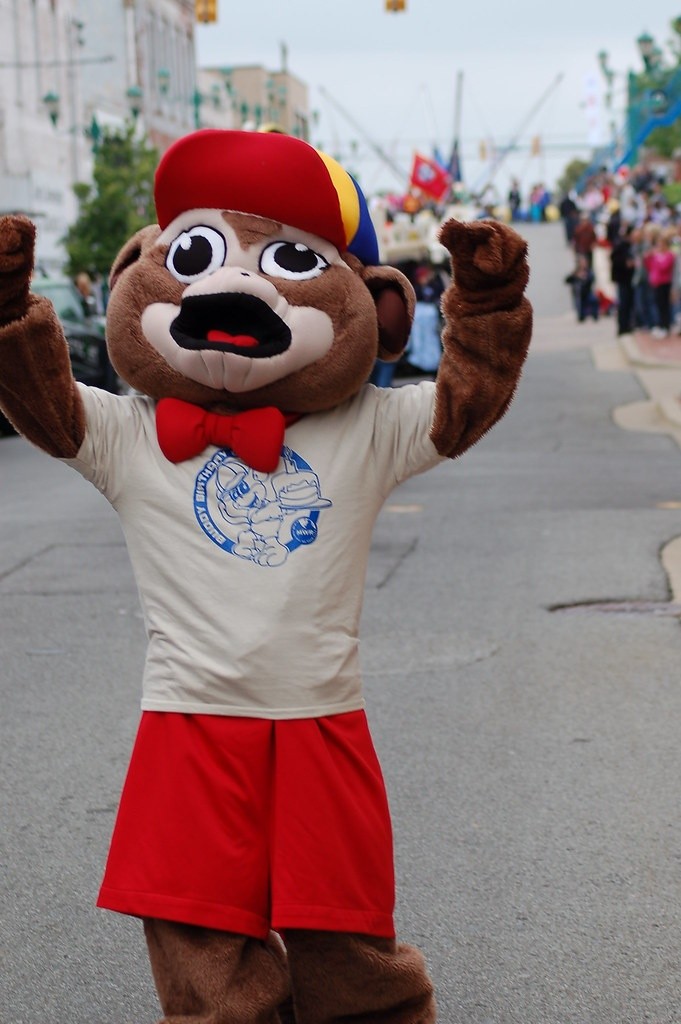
(0, 128), (536, 1024)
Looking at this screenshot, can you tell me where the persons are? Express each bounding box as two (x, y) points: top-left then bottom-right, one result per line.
(368, 149), (681, 373)
